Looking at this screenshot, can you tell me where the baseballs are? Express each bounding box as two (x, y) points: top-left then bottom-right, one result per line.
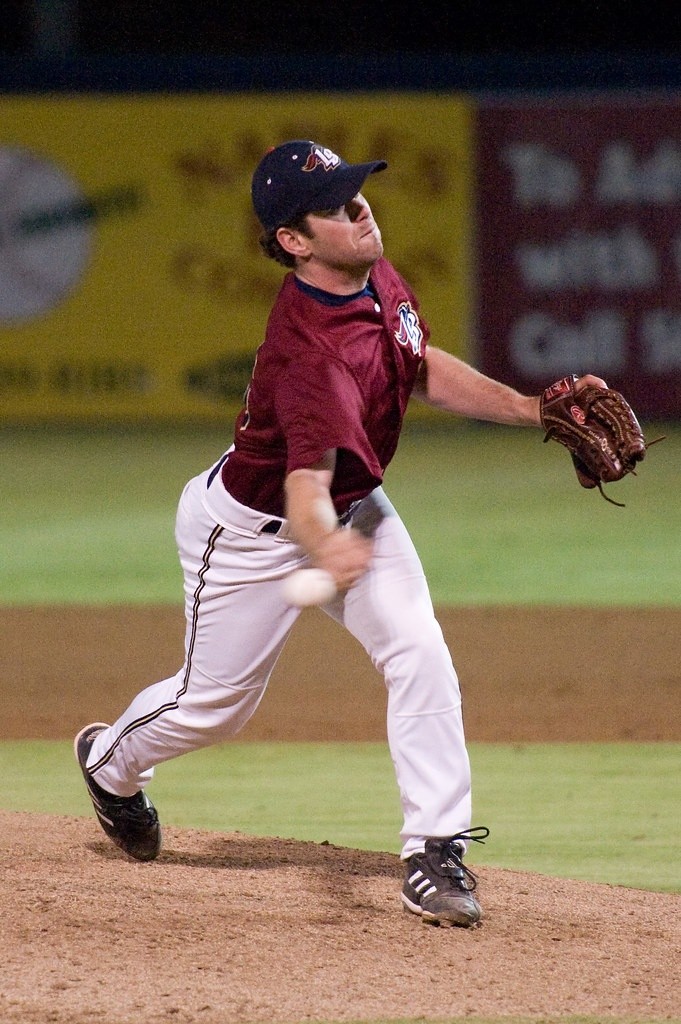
(277, 568), (334, 607)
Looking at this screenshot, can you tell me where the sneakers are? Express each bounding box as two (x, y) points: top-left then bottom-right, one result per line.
(399, 826), (490, 929)
(72, 722), (163, 862)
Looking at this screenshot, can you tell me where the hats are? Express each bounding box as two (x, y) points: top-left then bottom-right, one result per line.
(250, 141), (387, 230)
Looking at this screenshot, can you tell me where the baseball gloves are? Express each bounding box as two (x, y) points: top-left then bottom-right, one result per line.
(539, 372), (646, 490)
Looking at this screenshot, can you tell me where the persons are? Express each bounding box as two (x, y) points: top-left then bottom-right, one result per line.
(73, 137), (608, 930)
